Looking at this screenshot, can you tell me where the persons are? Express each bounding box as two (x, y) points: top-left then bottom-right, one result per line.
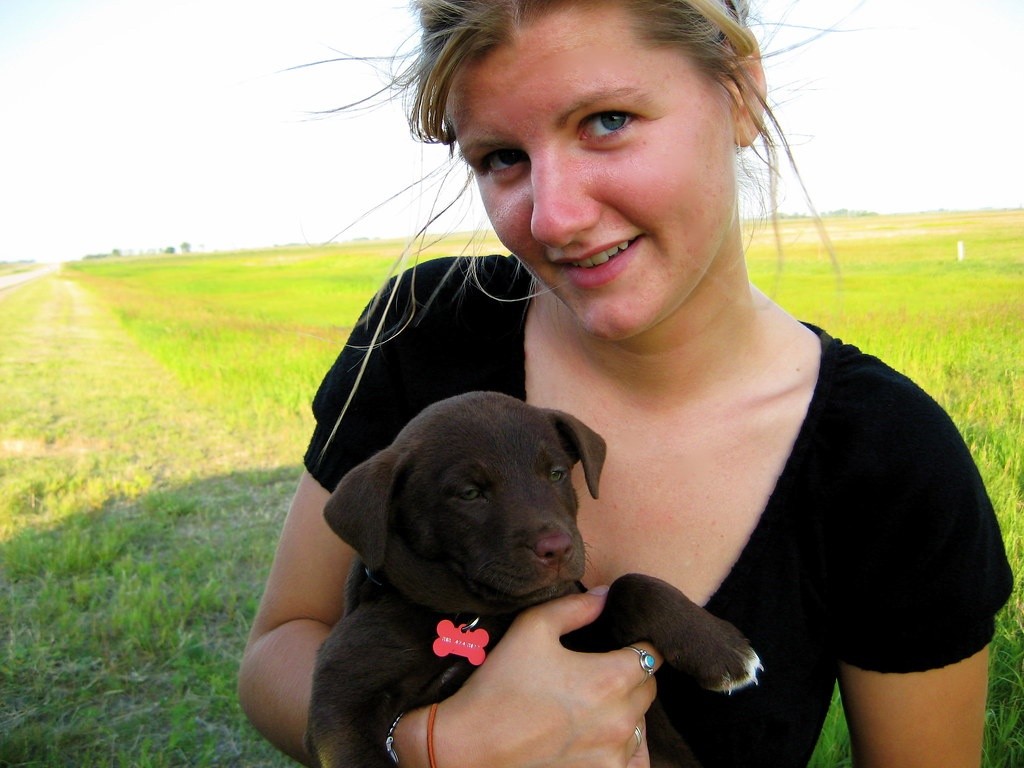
(233, 0), (1016, 768)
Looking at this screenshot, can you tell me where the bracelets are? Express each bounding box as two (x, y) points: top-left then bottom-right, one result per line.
(426, 703), (438, 768)
(383, 711), (405, 768)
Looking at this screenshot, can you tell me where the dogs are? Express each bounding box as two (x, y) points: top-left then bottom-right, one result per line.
(300, 389), (767, 768)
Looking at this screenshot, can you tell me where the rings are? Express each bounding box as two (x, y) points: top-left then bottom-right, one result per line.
(634, 724), (643, 750)
(619, 644), (656, 690)
